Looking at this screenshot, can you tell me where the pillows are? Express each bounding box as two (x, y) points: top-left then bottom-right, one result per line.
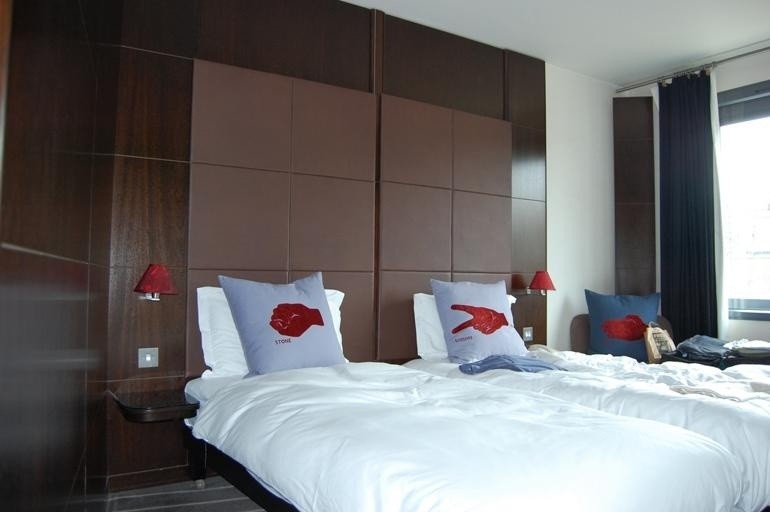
(585, 289), (661, 364)
(413, 292), (530, 364)
(217, 272), (347, 379)
(431, 278), (528, 364)
(196, 285), (345, 380)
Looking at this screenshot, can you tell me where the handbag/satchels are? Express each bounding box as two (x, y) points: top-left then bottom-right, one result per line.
(643, 321), (677, 364)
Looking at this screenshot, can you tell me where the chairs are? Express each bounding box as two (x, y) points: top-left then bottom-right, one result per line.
(569, 314), (674, 365)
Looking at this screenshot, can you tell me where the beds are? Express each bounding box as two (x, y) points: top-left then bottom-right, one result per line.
(183, 343), (769, 512)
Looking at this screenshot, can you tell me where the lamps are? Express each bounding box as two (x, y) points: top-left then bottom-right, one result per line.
(526, 269), (556, 295)
(511, 274), (527, 295)
(134, 263), (178, 301)
